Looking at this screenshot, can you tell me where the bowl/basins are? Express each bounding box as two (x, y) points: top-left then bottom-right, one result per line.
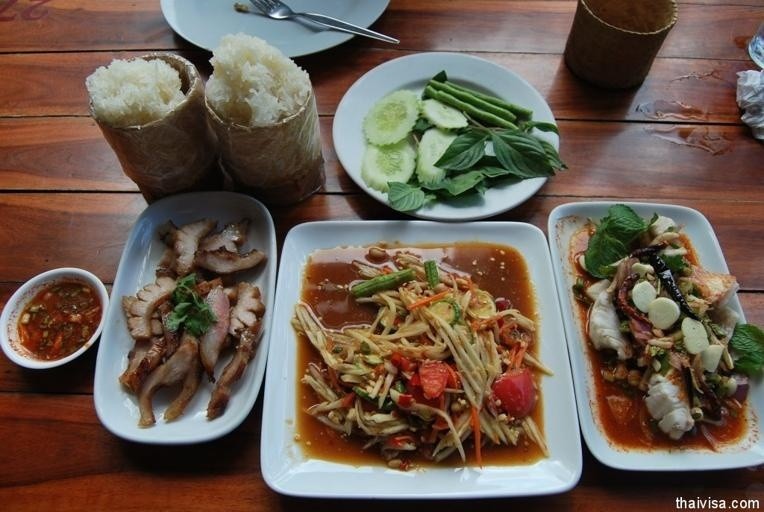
(1, 267), (109, 368)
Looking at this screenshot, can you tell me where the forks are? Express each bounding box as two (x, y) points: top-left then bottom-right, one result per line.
(252, 0), (401, 45)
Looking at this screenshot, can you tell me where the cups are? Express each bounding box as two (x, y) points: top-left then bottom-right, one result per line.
(87, 51), (205, 198)
(748, 21), (764, 69)
(204, 84), (325, 204)
(563, 0), (679, 94)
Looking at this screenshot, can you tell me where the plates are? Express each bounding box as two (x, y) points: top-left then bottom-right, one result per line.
(548, 201), (763, 472)
(93, 190), (278, 446)
(332, 50), (558, 222)
(260, 221), (582, 500)
(160, 1), (390, 58)
